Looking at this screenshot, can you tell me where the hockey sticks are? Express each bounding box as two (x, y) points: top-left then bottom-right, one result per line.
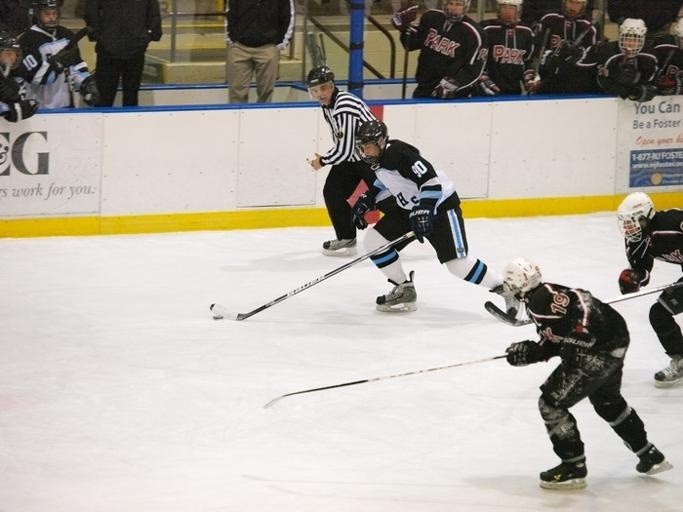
(485, 282), (682, 327)
(208, 230), (414, 321)
(262, 355), (511, 412)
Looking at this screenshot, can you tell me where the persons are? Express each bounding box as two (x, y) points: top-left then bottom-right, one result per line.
(224, 0), (295, 104)
(617, 194), (683, 384)
(501, 263), (664, 489)
(306, 66), (389, 254)
(84, 1), (163, 107)
(350, 119), (522, 317)
(0, 36), (37, 121)
(399, 0), (683, 102)
(19, 1), (99, 111)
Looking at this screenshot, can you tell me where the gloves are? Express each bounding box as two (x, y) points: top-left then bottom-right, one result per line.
(507, 341), (543, 367)
(80, 74), (100, 106)
(522, 69), (542, 91)
(619, 269), (641, 295)
(545, 52), (577, 81)
(655, 74), (680, 96)
(474, 75), (500, 97)
(392, 2), (418, 30)
(429, 75), (464, 98)
(410, 205), (434, 244)
(4, 99), (39, 123)
(553, 38), (583, 67)
(613, 64), (639, 100)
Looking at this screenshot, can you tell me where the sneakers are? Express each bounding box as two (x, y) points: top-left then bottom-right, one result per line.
(376, 271), (418, 306)
(323, 238), (356, 250)
(489, 271), (520, 319)
(540, 457), (587, 483)
(636, 445), (664, 473)
(654, 352), (682, 382)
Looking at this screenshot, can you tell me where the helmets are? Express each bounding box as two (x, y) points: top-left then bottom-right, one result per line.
(305, 66), (335, 101)
(619, 18), (648, 58)
(503, 259), (543, 303)
(671, 6), (683, 51)
(32, 1), (60, 32)
(615, 192), (656, 244)
(495, 0), (522, 29)
(0, 30), (24, 72)
(354, 120), (388, 170)
(441, 0), (472, 24)
(560, 0), (588, 22)
